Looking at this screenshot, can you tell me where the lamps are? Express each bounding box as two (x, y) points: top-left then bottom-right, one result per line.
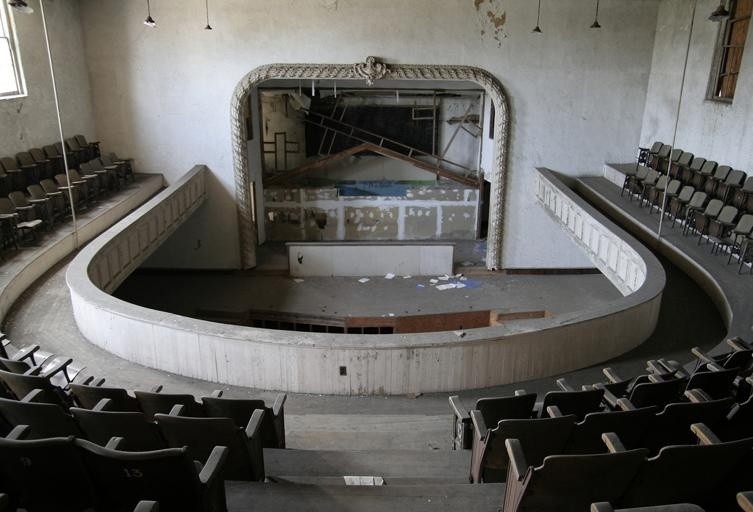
(530, 1), (543, 34)
(142, 1), (154, 27)
(589, 2), (603, 31)
(9, 1), (35, 19)
(202, 0), (212, 31)
(708, 2), (729, 26)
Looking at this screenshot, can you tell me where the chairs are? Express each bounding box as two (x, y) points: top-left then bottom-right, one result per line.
(446, 333), (753, 512)
(0, 133), (134, 264)
(619, 140), (751, 275)
(0, 327), (288, 512)
